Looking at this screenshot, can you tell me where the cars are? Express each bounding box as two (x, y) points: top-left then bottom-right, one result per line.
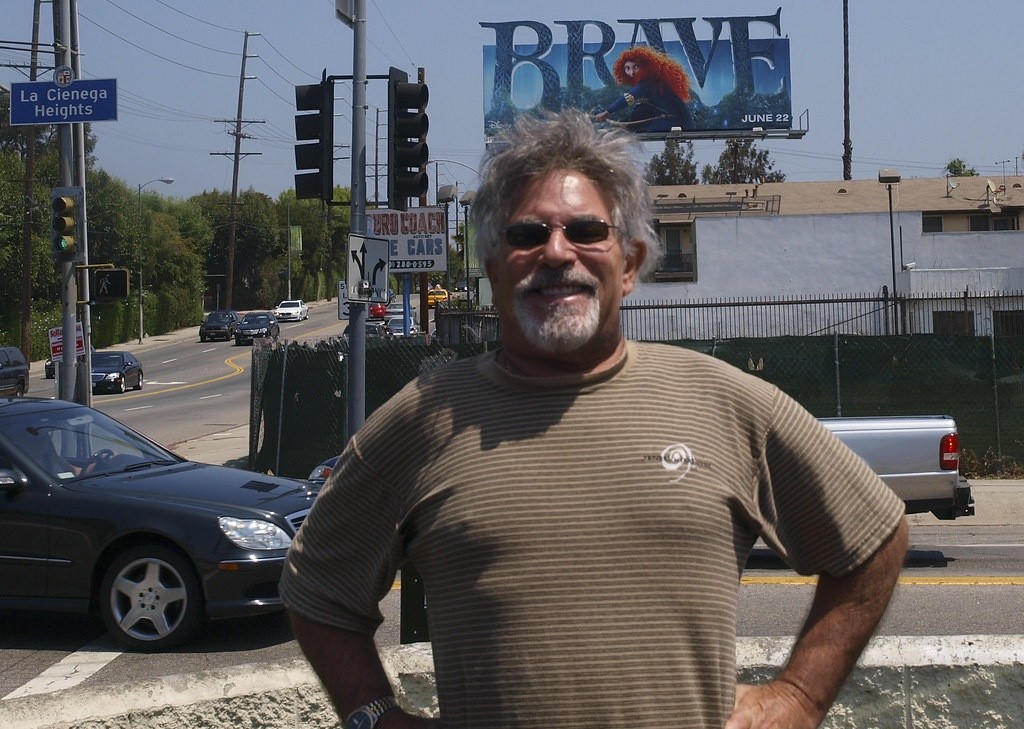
(44, 346), (95, 380)
(274, 299), (308, 323)
(384, 303), (417, 325)
(369, 303), (386, 320)
(455, 281), (468, 292)
(386, 317), (418, 338)
(199, 310), (241, 343)
(91, 350), (144, 396)
(428, 288), (449, 309)
(0, 393), (336, 655)
(234, 310), (280, 346)
(0, 346), (30, 398)
(342, 322), (388, 340)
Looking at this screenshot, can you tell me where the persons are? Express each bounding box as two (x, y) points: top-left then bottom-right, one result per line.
(275, 103), (908, 729)
(15, 424), (117, 480)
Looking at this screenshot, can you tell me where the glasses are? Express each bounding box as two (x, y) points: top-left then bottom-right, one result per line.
(497, 219), (629, 249)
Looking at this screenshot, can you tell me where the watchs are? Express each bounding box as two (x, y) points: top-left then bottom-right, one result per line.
(344, 695), (400, 729)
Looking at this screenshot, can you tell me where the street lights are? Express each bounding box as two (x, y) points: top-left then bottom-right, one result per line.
(135, 178), (175, 345)
(878, 167), (902, 335)
(460, 190), (477, 344)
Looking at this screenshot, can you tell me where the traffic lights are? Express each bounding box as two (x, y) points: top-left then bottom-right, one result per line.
(293, 67), (327, 202)
(387, 66), (429, 210)
(93, 268), (131, 302)
(49, 185), (85, 264)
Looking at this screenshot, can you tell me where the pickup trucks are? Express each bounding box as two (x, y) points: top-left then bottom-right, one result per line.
(307, 415), (977, 522)
